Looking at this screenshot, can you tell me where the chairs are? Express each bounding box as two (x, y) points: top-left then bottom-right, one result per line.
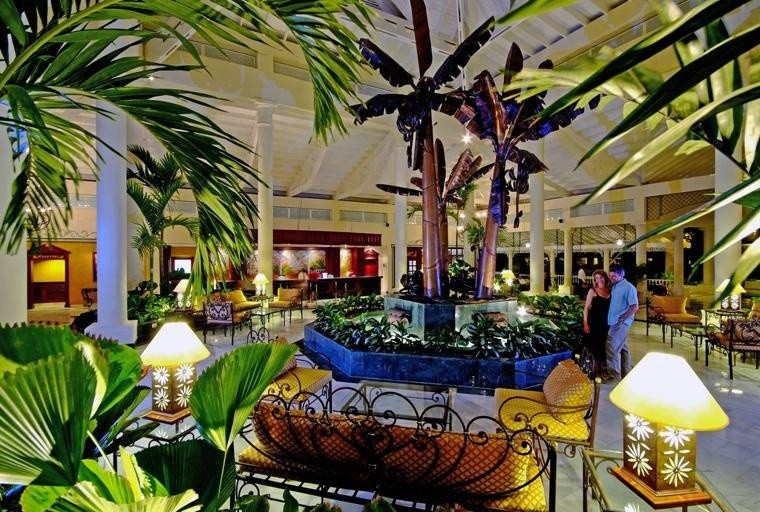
(705, 309), (760, 380)
(268, 287), (303, 324)
(646, 295), (702, 348)
(245, 328), (333, 414)
(494, 344), (602, 500)
(203, 300), (252, 345)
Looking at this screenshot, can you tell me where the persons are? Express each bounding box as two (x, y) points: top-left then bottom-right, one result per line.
(605, 265), (640, 385)
(577, 266), (587, 300)
(298, 267), (308, 279)
(583, 268), (612, 380)
(306, 267), (320, 300)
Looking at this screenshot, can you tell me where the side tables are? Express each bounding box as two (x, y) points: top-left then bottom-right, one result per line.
(122, 416), (203, 449)
(705, 309), (747, 356)
(164, 308), (207, 343)
(582, 448), (738, 512)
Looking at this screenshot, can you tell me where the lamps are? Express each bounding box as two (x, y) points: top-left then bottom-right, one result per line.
(173, 279), (193, 311)
(609, 352), (730, 509)
(501, 270), (516, 286)
(252, 273), (270, 296)
(140, 322), (211, 423)
(715, 279), (747, 313)
(578, 228), (585, 279)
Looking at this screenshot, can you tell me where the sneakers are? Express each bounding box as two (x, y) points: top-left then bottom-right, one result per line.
(601, 371), (620, 384)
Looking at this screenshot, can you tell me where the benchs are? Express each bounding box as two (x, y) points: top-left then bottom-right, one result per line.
(211, 287), (261, 331)
(235, 386), (557, 512)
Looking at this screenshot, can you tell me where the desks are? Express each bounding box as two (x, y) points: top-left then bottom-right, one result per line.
(81, 288), (97, 306)
